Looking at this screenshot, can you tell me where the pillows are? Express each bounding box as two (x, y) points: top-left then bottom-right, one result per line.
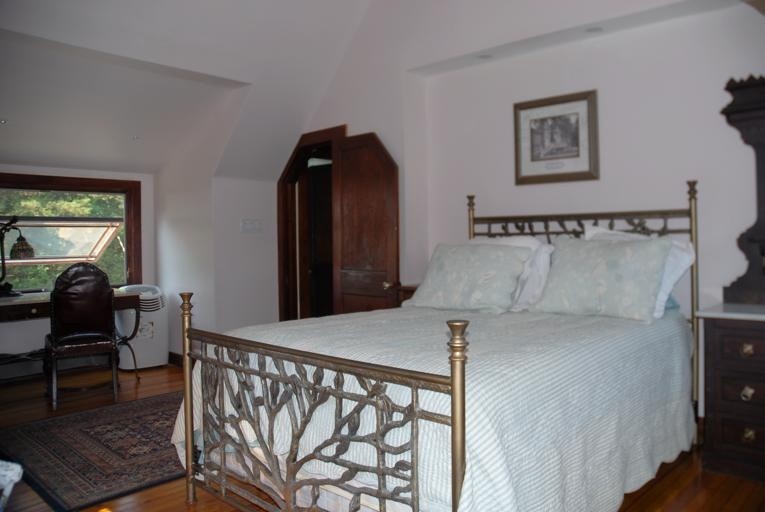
(403, 229), (693, 323)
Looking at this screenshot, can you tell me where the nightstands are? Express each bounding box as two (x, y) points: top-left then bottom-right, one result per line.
(699, 318), (765, 482)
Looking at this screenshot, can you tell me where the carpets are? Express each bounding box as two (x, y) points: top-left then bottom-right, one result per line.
(0, 388), (200, 512)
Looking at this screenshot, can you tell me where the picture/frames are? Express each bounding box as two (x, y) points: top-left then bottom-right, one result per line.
(510, 88), (601, 184)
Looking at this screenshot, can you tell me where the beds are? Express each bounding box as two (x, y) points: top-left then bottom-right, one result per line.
(179, 180), (700, 512)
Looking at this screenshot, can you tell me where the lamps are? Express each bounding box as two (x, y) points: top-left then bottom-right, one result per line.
(0, 213), (33, 307)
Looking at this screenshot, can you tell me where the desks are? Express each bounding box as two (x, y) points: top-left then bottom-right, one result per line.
(0, 291), (141, 384)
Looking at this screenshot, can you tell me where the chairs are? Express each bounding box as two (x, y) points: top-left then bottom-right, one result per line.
(43, 261), (122, 413)
